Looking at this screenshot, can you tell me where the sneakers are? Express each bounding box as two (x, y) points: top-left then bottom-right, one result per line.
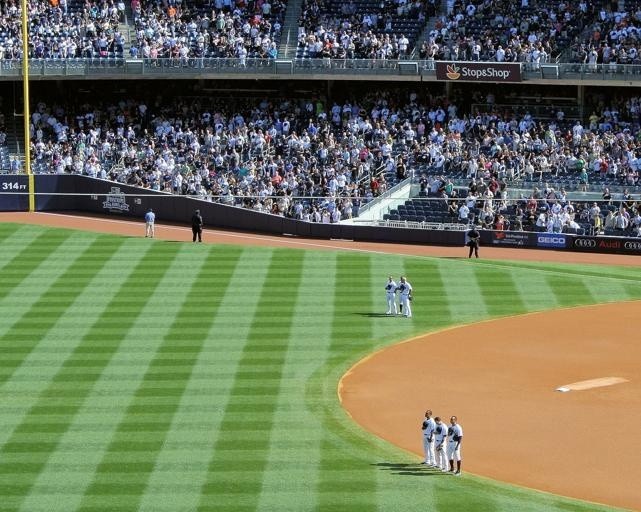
(384, 308), (413, 319)
(418, 458), (464, 478)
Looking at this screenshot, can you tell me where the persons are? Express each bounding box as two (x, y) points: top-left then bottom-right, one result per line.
(430, 416), (449, 473)
(385, 276), (413, 318)
(0, 0), (641, 242)
(467, 224), (480, 259)
(446, 416), (463, 476)
(421, 410), (437, 467)
